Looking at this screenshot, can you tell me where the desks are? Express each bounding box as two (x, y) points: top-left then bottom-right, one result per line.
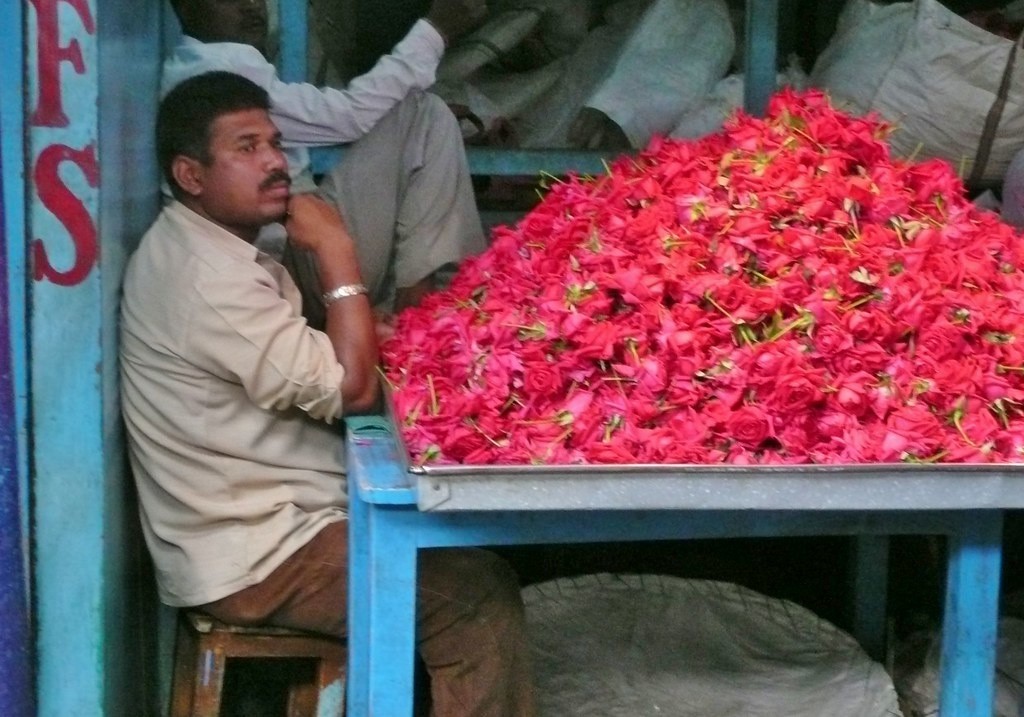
(342, 418), (1003, 716)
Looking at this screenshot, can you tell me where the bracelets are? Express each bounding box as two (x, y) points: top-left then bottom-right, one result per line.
(322, 284), (368, 307)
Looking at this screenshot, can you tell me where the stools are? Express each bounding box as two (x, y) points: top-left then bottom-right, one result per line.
(167, 609), (348, 715)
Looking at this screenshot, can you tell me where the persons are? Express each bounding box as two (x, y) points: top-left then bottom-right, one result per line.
(119, 70), (535, 717)
(162, 1), (487, 332)
